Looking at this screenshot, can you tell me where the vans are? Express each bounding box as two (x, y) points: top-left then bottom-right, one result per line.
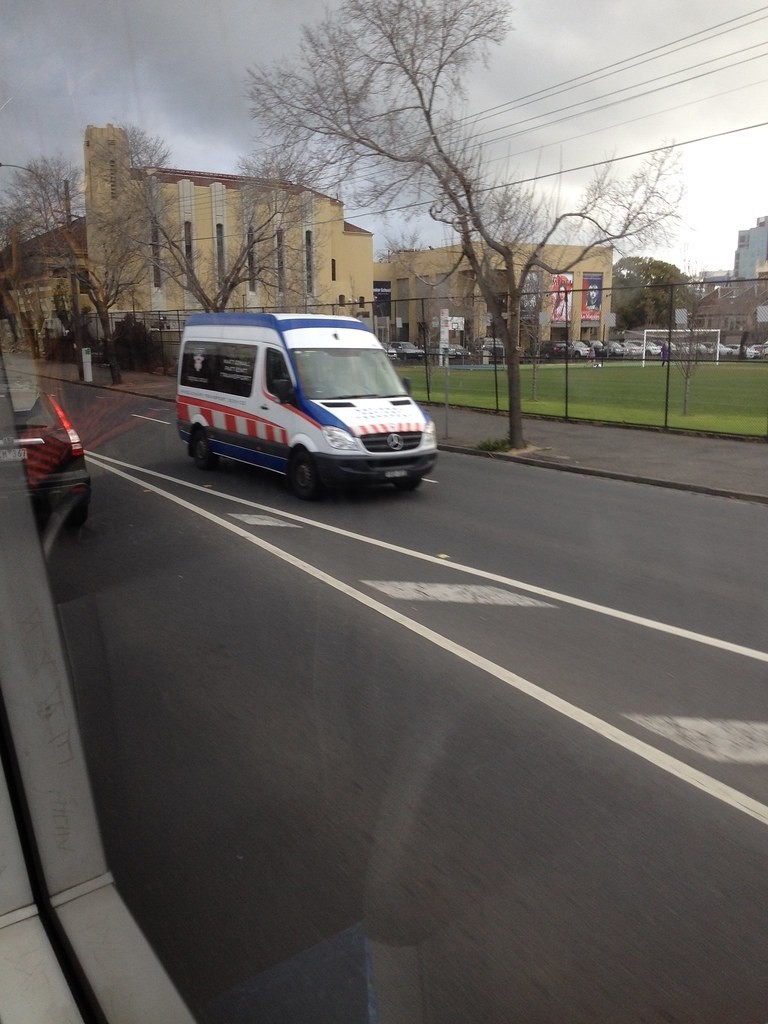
(177, 311), (439, 503)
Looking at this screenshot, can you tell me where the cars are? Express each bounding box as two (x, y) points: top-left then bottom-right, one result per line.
(383, 337), (768, 364)
(1, 379), (94, 530)
